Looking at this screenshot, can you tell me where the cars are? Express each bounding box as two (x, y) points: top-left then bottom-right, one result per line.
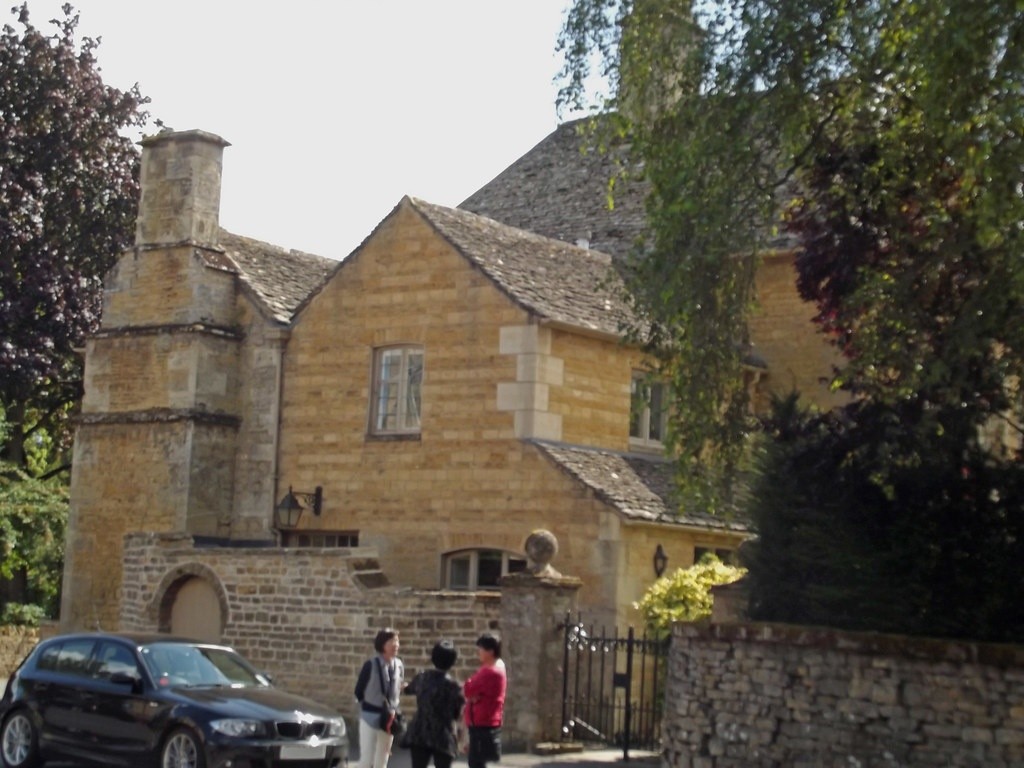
(0, 634), (350, 768)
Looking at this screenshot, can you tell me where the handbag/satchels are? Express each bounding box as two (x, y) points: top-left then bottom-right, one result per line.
(379, 709), (403, 736)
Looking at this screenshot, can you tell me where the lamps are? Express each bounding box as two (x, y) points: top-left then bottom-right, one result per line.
(654, 544), (667, 578)
(276, 485), (321, 527)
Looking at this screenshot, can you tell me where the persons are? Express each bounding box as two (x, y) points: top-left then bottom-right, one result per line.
(401, 637), (468, 767)
(353, 628), (403, 767)
(461, 632), (509, 768)
